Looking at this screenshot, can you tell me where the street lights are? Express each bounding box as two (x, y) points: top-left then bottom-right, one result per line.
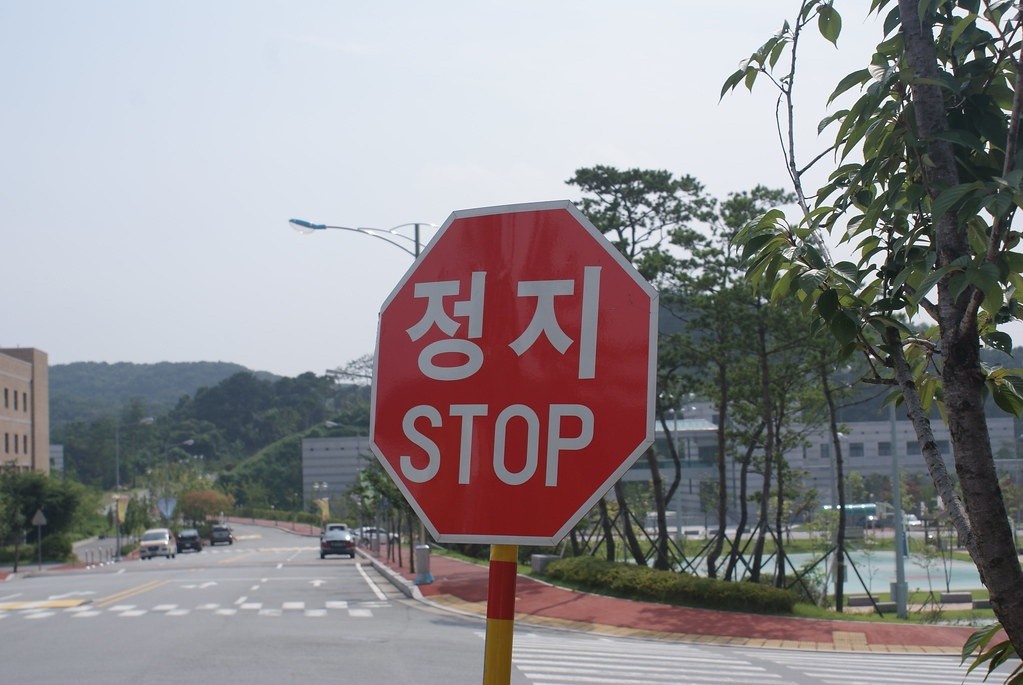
(114, 417), (155, 561)
(286, 217), (438, 587)
(163, 438), (197, 479)
(662, 393), (696, 532)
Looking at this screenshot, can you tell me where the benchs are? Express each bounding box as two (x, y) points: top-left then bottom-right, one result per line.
(531, 554), (560, 574)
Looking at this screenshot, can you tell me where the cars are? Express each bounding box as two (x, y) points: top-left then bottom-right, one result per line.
(211, 525), (234, 546)
(354, 527), (395, 544)
(175, 528), (203, 553)
(320, 523), (349, 538)
(320, 529), (355, 560)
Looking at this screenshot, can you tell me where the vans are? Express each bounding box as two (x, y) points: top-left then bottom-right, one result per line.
(139, 528), (177, 560)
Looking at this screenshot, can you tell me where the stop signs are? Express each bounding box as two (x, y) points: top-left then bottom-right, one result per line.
(369, 197), (660, 549)
